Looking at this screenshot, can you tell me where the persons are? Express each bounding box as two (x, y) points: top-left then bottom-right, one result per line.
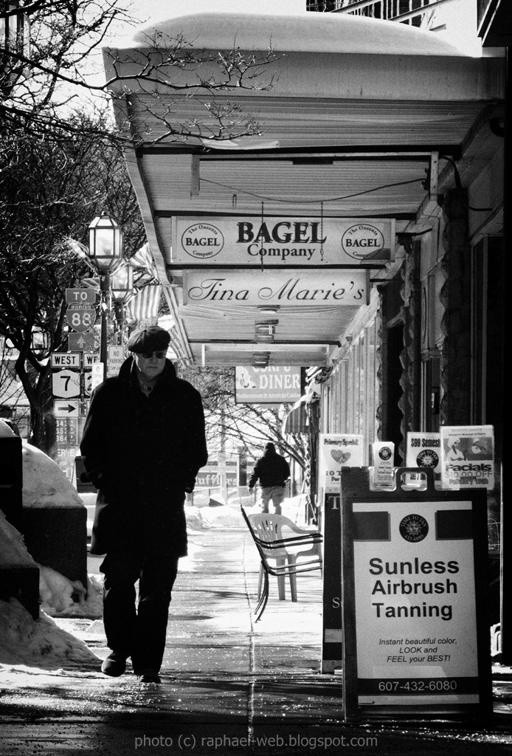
(447, 436), (464, 461)
(78, 326), (208, 684)
(248, 442), (290, 533)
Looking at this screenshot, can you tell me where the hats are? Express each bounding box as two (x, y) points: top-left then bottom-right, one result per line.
(264, 442), (275, 450)
(127, 326), (171, 353)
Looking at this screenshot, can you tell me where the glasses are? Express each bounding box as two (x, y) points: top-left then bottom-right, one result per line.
(140, 351), (166, 359)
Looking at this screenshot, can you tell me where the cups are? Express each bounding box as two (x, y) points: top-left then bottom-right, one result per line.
(367, 465), (429, 491)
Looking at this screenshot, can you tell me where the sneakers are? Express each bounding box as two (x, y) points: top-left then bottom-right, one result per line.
(101, 648), (129, 677)
(139, 672), (161, 683)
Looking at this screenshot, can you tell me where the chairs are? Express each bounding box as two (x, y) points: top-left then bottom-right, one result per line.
(240, 504), (325, 620)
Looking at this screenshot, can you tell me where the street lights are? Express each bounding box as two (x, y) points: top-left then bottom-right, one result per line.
(87, 210), (125, 380)
(109, 254), (134, 345)
